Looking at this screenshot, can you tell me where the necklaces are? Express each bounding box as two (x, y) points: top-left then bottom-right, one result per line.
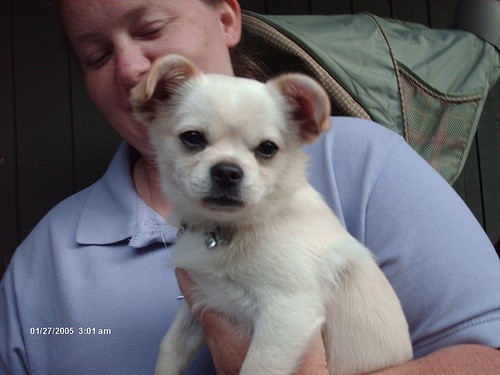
(139, 157), (185, 300)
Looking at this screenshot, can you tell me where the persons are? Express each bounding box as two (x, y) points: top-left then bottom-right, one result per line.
(0, 0), (500, 374)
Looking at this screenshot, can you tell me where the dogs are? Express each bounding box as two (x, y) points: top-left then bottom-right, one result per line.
(129, 53), (413, 375)
